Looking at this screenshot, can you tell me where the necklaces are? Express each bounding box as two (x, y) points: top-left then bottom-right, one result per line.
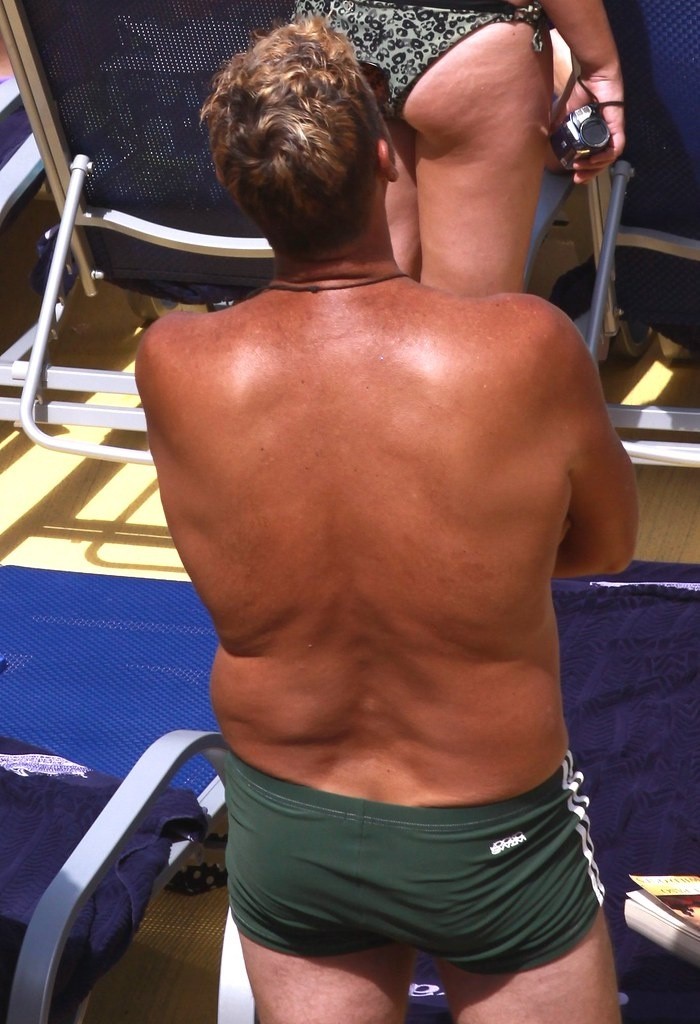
(245, 272), (407, 301)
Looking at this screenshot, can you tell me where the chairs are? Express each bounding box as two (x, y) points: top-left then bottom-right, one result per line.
(0, 0), (700, 468)
(0, 656), (225, 1024)
(214, 559), (700, 1024)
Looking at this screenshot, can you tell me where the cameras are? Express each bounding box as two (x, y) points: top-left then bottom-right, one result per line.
(549, 102), (610, 171)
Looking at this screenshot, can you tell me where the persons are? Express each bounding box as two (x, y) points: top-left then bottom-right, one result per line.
(134, 17), (640, 1024)
(288, 0), (626, 299)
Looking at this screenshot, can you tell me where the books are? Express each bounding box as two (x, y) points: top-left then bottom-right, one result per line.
(624, 875), (700, 968)
